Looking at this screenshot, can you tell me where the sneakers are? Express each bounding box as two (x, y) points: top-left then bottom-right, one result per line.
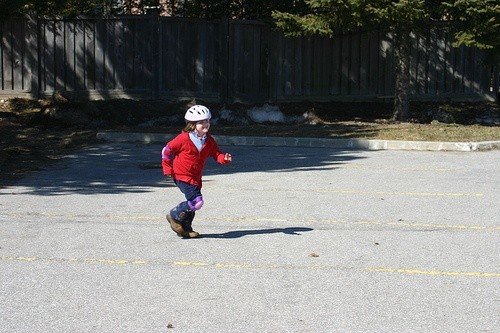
(177, 229), (199, 238)
(166, 214), (184, 234)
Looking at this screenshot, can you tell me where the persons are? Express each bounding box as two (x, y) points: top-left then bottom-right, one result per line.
(161, 105), (231, 239)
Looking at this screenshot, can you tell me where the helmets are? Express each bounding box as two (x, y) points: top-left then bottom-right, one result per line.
(185, 105), (211, 121)
